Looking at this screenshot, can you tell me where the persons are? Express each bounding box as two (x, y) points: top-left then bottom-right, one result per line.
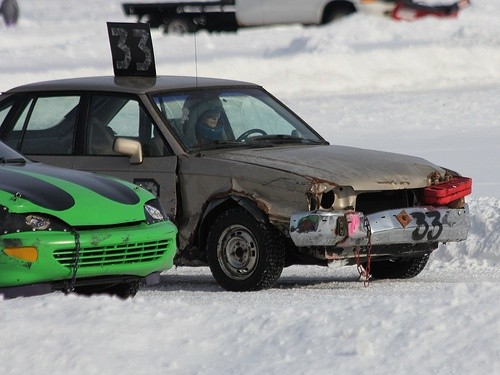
(194, 100), (230, 144)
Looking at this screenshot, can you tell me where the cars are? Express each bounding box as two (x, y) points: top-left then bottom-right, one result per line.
(0, 141), (179, 303)
(123, 0), (359, 35)
(1, 76), (469, 294)
